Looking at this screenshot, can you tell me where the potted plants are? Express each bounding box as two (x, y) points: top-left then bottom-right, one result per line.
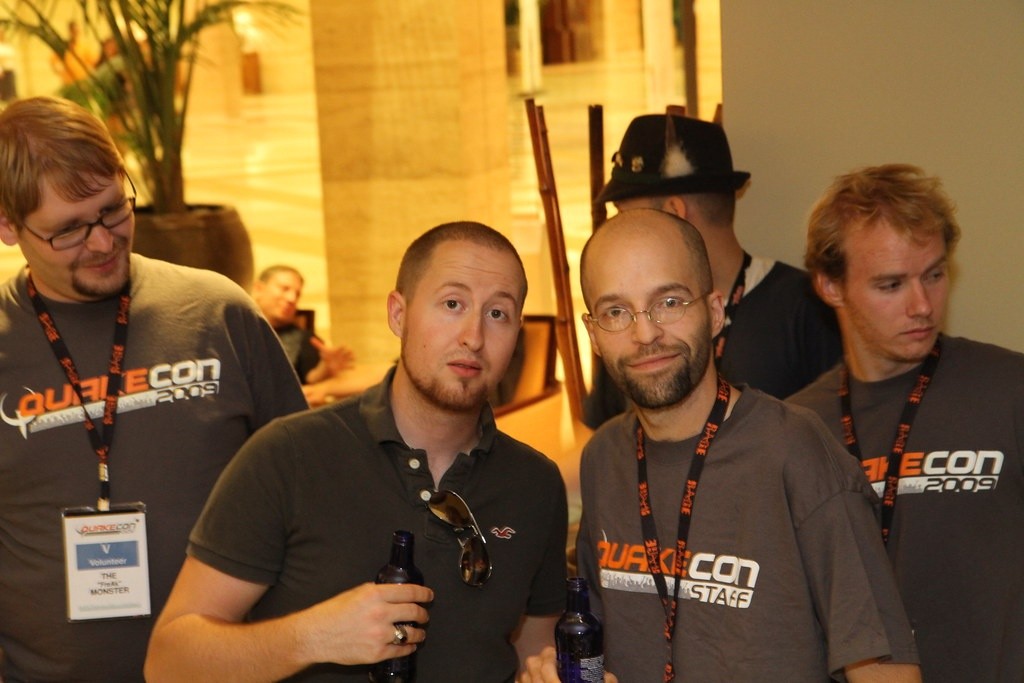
(0, 0), (311, 297)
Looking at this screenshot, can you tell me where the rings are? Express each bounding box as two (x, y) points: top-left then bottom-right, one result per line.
(391, 625), (407, 645)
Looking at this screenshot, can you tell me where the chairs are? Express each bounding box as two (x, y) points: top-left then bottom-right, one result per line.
(493, 315), (563, 471)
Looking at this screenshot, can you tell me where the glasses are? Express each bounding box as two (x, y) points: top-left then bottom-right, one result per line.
(585, 290), (715, 334)
(425, 488), (492, 586)
(19, 169), (137, 251)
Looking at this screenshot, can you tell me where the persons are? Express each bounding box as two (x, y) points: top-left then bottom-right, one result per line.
(0, 96), (309, 683)
(144, 221), (568, 683)
(782, 164), (1024, 683)
(579, 115), (845, 430)
(252, 265), (354, 386)
(515, 208), (921, 683)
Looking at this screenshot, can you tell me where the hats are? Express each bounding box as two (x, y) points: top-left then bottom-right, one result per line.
(592, 115), (751, 203)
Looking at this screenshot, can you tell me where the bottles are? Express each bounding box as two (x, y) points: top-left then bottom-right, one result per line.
(369, 529), (425, 683)
(555, 577), (606, 683)
